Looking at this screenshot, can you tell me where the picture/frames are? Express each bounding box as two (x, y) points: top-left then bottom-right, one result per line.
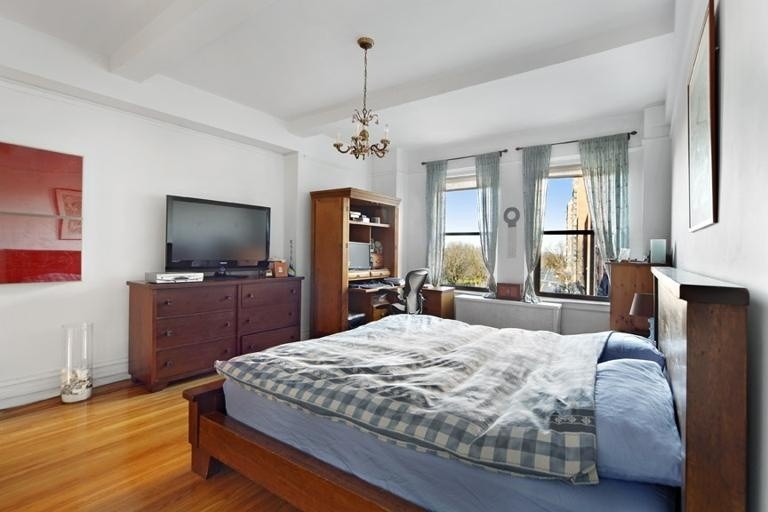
(55, 187), (82, 239)
(683, 1), (718, 233)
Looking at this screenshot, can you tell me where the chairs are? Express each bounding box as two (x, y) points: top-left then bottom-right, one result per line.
(375, 268), (429, 317)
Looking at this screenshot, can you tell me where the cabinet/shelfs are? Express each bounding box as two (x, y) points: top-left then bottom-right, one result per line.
(605, 260), (671, 335)
(310, 187), (402, 337)
(126, 275), (305, 392)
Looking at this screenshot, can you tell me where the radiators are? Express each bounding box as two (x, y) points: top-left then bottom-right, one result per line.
(454, 294), (562, 335)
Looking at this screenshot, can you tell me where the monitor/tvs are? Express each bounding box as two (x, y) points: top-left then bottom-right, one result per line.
(348, 242), (370, 271)
(165, 195), (271, 280)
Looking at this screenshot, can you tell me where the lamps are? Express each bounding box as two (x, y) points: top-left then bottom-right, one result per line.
(629, 292), (655, 340)
(333, 38), (393, 160)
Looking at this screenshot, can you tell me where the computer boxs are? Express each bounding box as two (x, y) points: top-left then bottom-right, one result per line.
(348, 312), (366, 330)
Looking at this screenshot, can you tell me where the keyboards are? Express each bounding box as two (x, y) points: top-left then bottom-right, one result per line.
(360, 283), (385, 289)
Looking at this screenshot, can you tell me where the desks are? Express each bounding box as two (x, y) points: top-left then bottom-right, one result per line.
(349, 282), (455, 321)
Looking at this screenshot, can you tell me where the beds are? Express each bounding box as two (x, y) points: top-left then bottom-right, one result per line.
(183, 265), (749, 511)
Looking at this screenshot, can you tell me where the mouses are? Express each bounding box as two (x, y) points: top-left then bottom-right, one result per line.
(389, 282), (393, 287)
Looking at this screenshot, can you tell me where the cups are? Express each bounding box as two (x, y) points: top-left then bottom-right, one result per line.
(370, 217), (381, 224)
(650, 239), (666, 264)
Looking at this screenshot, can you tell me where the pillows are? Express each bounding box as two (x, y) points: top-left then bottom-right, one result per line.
(603, 331), (666, 370)
(595, 359), (683, 488)
